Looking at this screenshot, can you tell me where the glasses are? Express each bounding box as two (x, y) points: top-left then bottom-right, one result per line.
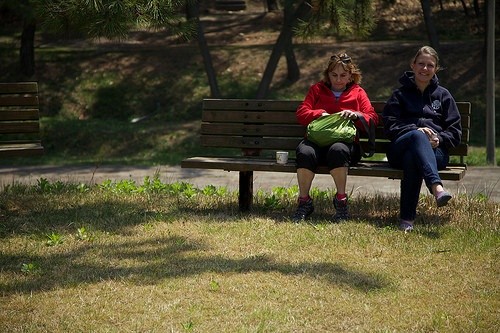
(331, 55), (351, 63)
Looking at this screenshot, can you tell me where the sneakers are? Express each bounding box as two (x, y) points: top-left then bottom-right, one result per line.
(333, 192), (352, 224)
(291, 198), (315, 223)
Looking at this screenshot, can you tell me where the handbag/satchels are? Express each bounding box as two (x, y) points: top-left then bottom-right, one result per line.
(304, 113), (360, 148)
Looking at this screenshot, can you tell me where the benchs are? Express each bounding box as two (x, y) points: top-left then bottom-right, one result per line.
(0, 82), (45, 156)
(180, 99), (471, 216)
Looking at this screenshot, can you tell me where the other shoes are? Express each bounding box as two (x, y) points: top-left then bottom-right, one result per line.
(399, 220), (414, 232)
(436, 193), (452, 207)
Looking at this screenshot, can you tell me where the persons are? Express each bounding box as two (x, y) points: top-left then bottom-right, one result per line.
(382, 45), (463, 232)
(294, 50), (379, 224)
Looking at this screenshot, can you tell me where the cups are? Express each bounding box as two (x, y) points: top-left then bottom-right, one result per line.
(427, 133), (438, 148)
(276, 151), (289, 164)
